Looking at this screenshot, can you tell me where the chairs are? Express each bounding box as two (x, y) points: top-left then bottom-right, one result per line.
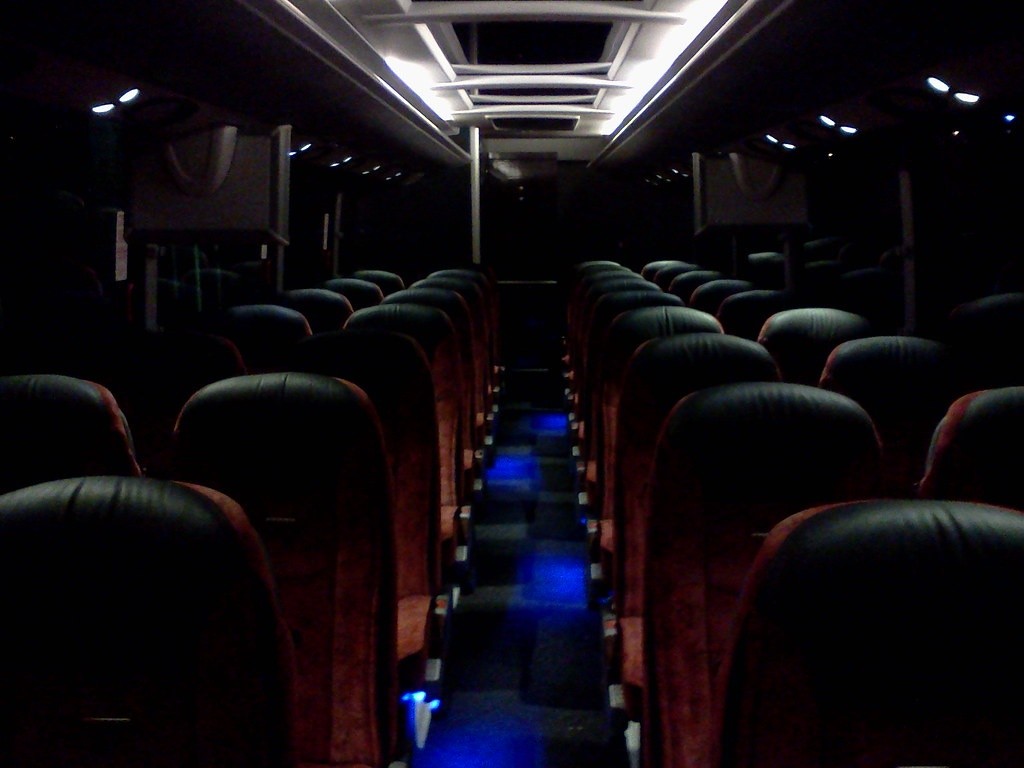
(0, 249), (1024, 768)
